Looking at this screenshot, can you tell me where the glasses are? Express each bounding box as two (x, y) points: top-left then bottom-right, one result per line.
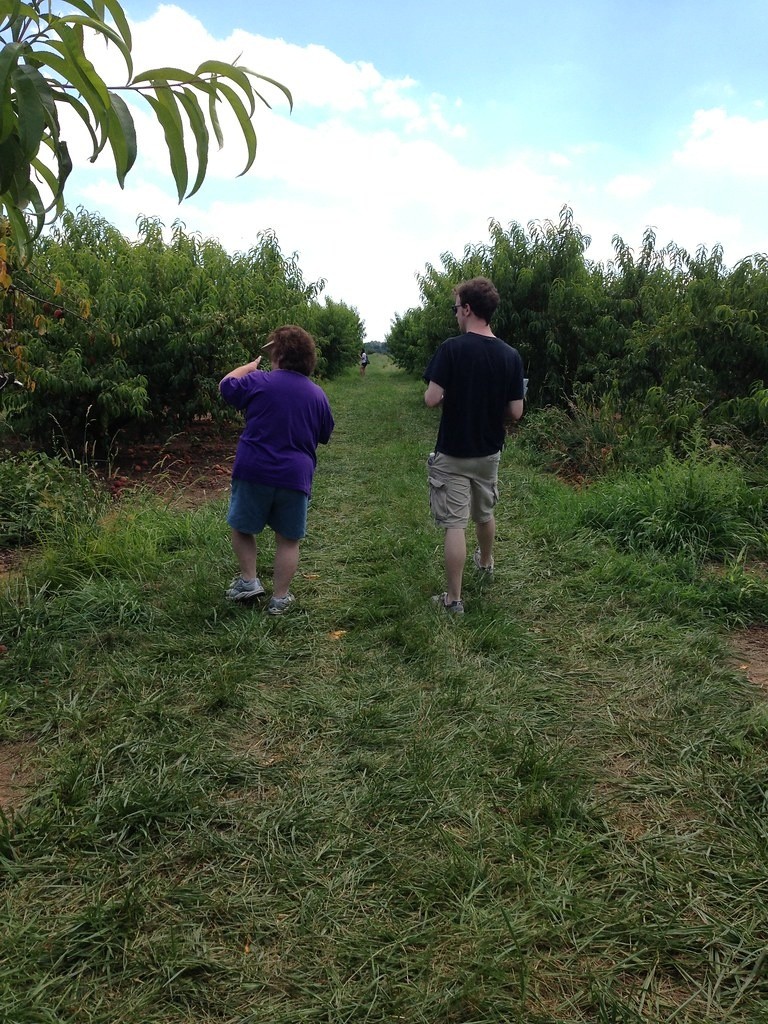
(451, 303), (463, 313)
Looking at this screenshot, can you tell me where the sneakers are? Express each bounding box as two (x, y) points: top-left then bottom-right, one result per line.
(473, 545), (495, 575)
(430, 592), (465, 617)
(268, 593), (296, 614)
(224, 577), (266, 600)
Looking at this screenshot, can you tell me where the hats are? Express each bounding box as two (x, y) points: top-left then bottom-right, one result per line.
(261, 338), (276, 352)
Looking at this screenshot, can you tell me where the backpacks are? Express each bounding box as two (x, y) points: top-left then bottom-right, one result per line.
(364, 356), (370, 364)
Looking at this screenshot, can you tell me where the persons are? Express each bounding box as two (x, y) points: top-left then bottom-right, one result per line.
(358, 348), (367, 376)
(219, 326), (334, 614)
(423, 276), (524, 617)
